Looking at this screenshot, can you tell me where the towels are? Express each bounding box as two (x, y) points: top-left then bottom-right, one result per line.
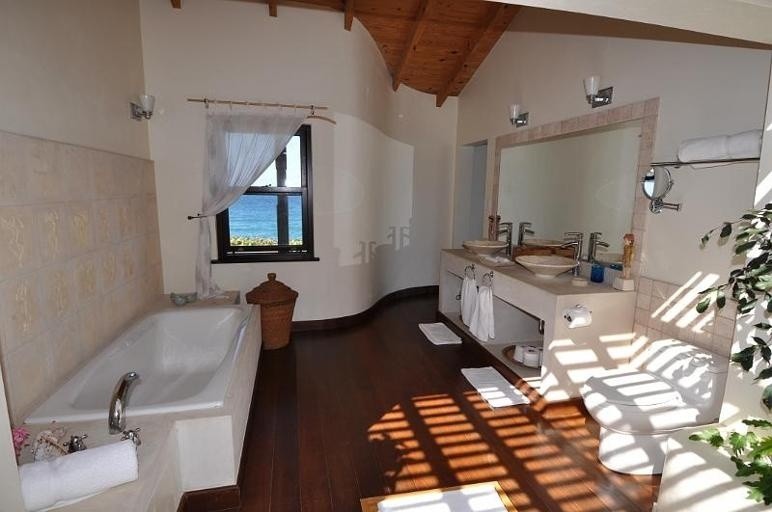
(460, 276), (476, 327)
(18, 440), (139, 511)
(469, 286), (495, 342)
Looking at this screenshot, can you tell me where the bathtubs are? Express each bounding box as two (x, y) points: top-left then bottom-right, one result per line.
(41, 303), (263, 491)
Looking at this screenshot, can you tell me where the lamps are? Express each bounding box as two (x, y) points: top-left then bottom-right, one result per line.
(506, 104), (530, 127)
(580, 74), (613, 110)
(128, 94), (155, 123)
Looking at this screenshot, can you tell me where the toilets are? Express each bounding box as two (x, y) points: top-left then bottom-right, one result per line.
(583, 337), (728, 475)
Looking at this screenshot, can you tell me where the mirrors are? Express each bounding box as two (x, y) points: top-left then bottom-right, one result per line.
(487, 96), (661, 291)
(642, 167), (680, 212)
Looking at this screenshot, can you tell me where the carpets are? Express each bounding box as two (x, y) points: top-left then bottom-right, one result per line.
(460, 366), (530, 408)
(360, 481), (518, 512)
(418, 322), (462, 346)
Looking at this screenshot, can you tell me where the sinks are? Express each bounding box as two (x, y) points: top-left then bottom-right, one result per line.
(515, 254), (580, 275)
(462, 240), (509, 254)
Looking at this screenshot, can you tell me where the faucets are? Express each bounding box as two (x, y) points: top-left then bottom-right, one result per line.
(496, 222), (512, 246)
(109, 371), (139, 434)
(518, 222), (535, 243)
(496, 246), (513, 256)
(588, 231), (609, 263)
(560, 231), (583, 270)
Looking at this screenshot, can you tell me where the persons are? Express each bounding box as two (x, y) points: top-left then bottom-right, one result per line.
(622, 234), (635, 279)
(488, 216), (495, 240)
